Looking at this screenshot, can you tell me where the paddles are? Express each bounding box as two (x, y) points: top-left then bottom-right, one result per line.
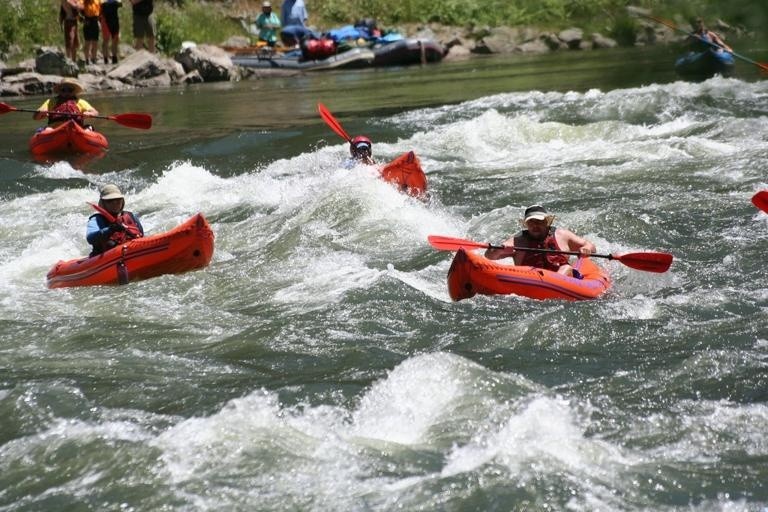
(752, 191), (768, 214)
(318, 103), (376, 164)
(426, 235), (672, 273)
(649, 17), (767, 76)
(0, 102), (152, 130)
(85, 200), (140, 240)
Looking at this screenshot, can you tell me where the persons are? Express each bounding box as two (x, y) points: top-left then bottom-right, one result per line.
(485, 204), (597, 278)
(33, 77), (99, 135)
(79, 0), (101, 65)
(340, 135), (375, 170)
(86, 184), (144, 259)
(681, 16), (732, 54)
(130, 0), (156, 53)
(256, 0), (320, 62)
(99, 0), (122, 64)
(59, 0), (85, 62)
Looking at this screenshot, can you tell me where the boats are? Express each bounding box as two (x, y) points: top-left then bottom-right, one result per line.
(32, 150), (106, 178)
(47, 212), (214, 288)
(229, 47), (375, 72)
(447, 246), (612, 305)
(30, 118), (109, 153)
(374, 38), (444, 67)
(673, 43), (735, 85)
(376, 150), (429, 201)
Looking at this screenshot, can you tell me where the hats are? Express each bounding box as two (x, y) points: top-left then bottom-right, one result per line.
(523, 205), (555, 228)
(54, 79), (83, 94)
(100, 185), (123, 200)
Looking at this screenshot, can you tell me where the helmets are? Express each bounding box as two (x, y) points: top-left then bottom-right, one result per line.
(350, 136), (371, 155)
